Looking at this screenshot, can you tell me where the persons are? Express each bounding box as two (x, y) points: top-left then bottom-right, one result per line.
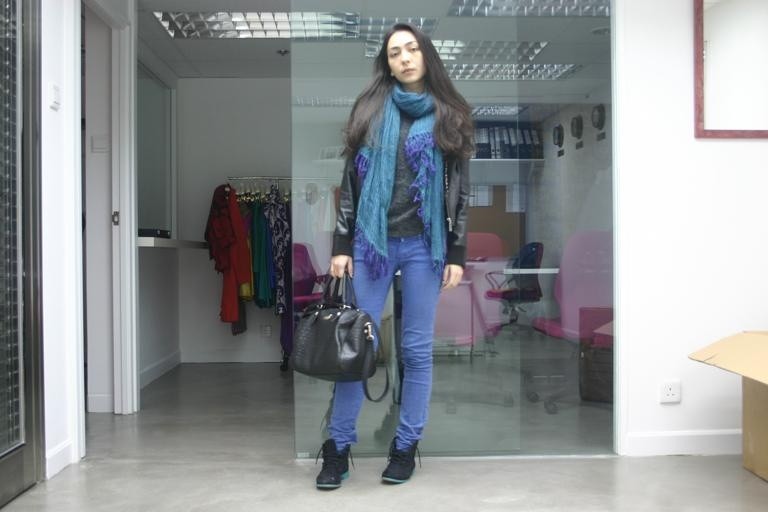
(315, 22), (471, 488)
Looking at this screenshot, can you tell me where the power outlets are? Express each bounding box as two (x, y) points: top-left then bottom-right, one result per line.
(658, 381), (681, 403)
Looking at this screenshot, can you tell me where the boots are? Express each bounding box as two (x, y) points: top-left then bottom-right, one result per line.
(315, 440), (355, 489)
(382, 438), (421, 485)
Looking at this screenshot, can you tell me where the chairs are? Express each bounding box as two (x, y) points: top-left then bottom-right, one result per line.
(277, 243), (334, 372)
(435, 229), (614, 413)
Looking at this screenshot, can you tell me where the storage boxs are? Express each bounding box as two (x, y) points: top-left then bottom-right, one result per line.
(688, 324), (768, 483)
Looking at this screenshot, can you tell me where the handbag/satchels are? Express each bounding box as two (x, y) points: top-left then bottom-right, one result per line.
(292, 273), (389, 403)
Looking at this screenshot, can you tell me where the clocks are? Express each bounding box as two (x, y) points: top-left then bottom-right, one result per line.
(553, 104), (605, 148)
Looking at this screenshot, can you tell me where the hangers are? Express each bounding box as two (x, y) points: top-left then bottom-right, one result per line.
(224, 175), (343, 205)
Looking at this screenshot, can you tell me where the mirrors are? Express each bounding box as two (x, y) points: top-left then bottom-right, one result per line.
(138, 63), (174, 238)
(692, 1), (768, 139)
